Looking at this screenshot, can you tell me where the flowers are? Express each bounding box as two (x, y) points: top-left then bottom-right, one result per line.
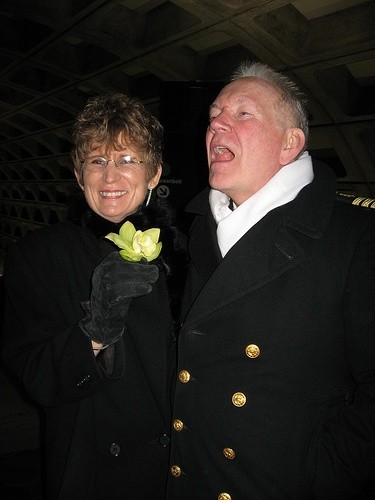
(106, 221), (162, 263)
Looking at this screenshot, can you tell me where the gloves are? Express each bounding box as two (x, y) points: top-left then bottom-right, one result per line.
(80, 252), (159, 345)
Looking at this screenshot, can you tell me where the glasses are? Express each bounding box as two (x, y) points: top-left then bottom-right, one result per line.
(80, 156), (147, 172)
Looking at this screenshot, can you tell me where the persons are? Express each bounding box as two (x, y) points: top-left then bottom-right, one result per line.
(0, 100), (188, 500)
(166, 60), (375, 500)
